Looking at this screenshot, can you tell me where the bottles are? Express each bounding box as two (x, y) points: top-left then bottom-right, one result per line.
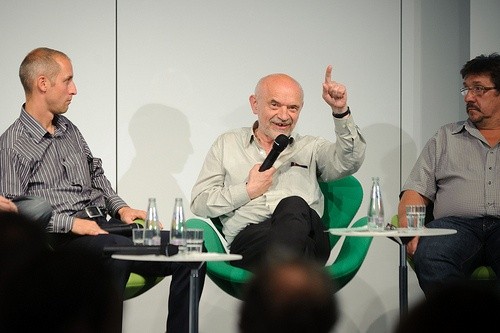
(367, 176), (384, 232)
(169, 198), (186, 252)
(143, 197), (161, 246)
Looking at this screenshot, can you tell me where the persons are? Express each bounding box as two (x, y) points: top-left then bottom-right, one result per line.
(389, 276), (500, 333)
(190, 65), (367, 276)
(238, 258), (340, 333)
(0, 47), (162, 289)
(399, 51), (500, 298)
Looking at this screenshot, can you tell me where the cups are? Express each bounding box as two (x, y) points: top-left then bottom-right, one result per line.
(186, 229), (204, 254)
(132, 228), (145, 245)
(406, 204), (426, 230)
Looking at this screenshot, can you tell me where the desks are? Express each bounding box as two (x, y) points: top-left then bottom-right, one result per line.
(111, 248), (244, 333)
(327, 225), (459, 324)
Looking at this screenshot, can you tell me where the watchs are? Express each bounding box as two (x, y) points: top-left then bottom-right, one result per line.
(333, 107), (351, 118)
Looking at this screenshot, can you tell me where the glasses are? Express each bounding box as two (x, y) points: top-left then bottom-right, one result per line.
(459, 85), (496, 96)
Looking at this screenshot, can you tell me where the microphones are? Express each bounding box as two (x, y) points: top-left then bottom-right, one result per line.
(259, 134), (289, 172)
(103, 244), (180, 256)
(101, 223), (144, 236)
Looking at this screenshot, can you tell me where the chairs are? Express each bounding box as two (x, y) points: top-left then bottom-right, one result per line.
(181, 169), (377, 303)
